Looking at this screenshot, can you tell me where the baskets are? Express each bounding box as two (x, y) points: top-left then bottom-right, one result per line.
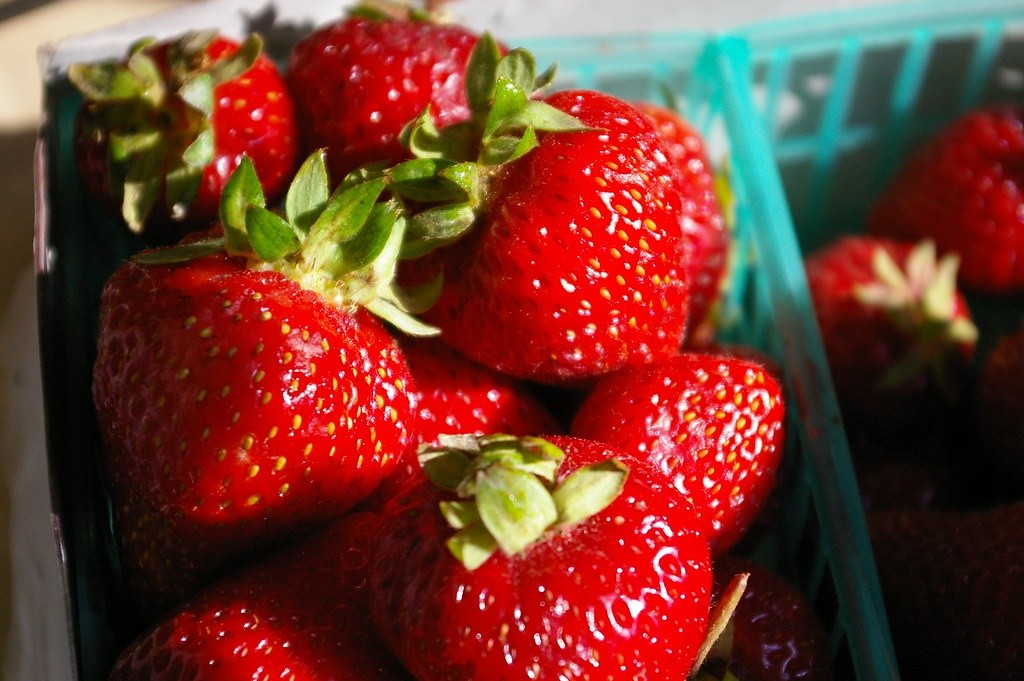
(712, 0), (1023, 680)
(35, 27), (897, 680)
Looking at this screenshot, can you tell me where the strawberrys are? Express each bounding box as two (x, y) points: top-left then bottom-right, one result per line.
(806, 105), (1023, 681)
(65, 3), (826, 681)
